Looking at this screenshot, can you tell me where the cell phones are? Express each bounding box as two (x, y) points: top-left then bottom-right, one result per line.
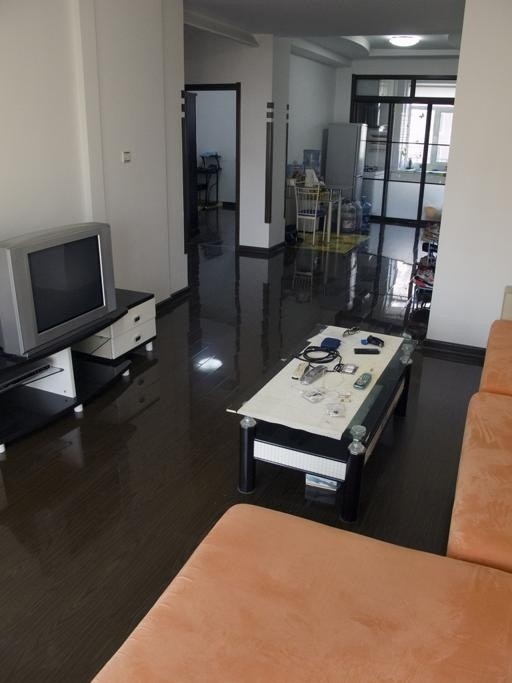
(354, 373), (372, 389)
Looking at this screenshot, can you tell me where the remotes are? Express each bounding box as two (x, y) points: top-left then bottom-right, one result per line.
(292, 363), (309, 380)
(301, 364), (327, 384)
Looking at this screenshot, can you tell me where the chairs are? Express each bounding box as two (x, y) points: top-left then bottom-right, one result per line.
(294, 180), (328, 247)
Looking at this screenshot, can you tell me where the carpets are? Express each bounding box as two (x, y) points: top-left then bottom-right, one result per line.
(291, 228), (371, 254)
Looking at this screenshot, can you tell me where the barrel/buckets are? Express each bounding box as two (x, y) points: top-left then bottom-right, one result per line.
(303, 148), (322, 174)
(353, 199), (363, 231)
(341, 199), (356, 232)
(361, 196), (372, 232)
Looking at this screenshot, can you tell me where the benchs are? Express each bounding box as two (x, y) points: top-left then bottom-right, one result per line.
(90, 284), (511, 683)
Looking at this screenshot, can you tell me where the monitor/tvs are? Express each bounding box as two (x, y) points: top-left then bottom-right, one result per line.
(0, 221), (117, 358)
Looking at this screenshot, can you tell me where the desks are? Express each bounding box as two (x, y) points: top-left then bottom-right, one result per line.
(2, 289), (159, 450)
(287, 184), (354, 243)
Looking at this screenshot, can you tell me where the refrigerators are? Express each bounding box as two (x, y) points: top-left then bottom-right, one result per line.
(324, 123), (368, 201)
